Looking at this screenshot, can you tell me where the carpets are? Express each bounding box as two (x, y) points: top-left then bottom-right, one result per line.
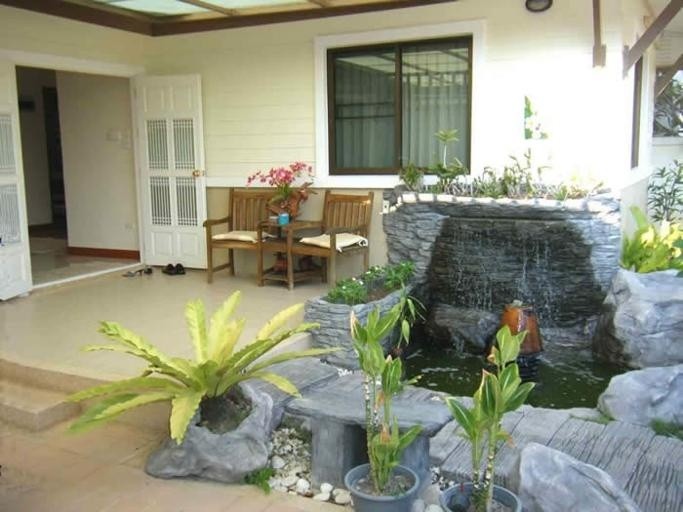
(28, 235), (66, 255)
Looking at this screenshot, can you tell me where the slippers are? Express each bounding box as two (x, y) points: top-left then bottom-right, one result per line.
(162, 263), (185, 275)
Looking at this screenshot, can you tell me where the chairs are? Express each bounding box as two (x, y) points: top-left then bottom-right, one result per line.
(202, 187), (373, 290)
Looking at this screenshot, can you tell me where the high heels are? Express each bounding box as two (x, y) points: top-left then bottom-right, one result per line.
(121, 264), (153, 278)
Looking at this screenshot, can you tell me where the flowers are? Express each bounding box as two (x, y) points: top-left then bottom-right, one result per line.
(325, 260), (417, 306)
(247, 162), (317, 214)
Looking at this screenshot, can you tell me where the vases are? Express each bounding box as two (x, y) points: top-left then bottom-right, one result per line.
(301, 280), (416, 370)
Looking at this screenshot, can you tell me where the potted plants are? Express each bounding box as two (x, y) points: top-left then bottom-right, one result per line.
(63, 289), (343, 484)
(439, 324), (535, 512)
(343, 283), (420, 512)
